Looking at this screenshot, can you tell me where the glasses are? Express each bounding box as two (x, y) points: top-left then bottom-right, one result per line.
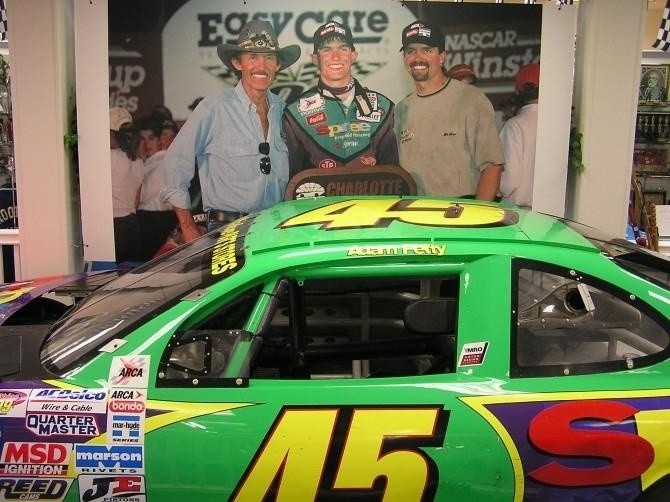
(258, 141), (272, 175)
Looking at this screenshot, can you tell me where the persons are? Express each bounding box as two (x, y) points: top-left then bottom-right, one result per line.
(645, 70), (661, 101)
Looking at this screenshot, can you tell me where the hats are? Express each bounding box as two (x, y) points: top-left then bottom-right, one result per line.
(108, 105), (135, 133)
(215, 19), (302, 75)
(497, 93), (522, 108)
(514, 62), (540, 92)
(445, 63), (479, 83)
(188, 96), (205, 111)
(399, 19), (447, 53)
(152, 104), (180, 132)
(312, 20), (356, 53)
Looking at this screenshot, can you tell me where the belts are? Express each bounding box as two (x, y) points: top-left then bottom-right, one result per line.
(208, 208), (249, 224)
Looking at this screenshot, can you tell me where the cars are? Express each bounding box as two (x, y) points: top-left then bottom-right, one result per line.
(0, 193), (666, 502)
